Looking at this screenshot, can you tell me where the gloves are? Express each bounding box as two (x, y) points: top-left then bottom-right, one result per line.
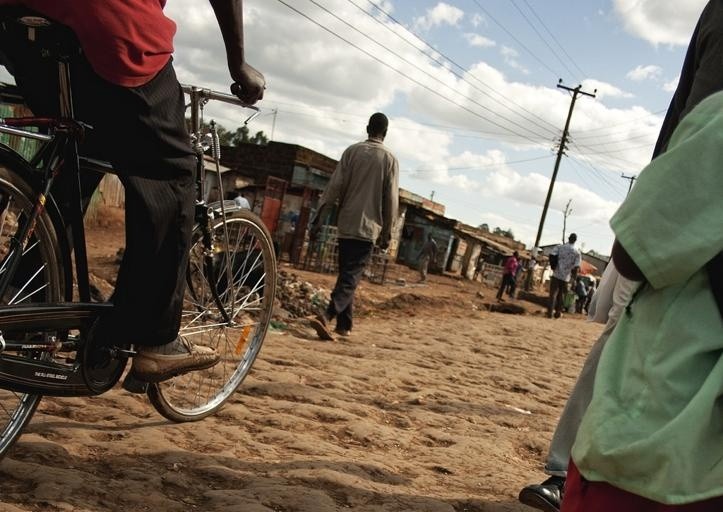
(588, 257), (642, 324)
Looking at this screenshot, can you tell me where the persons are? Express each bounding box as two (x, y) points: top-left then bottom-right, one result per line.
(520, 1), (723, 511)
(473, 255), (486, 281)
(0, 0), (265, 383)
(496, 250), (522, 301)
(309, 112), (399, 341)
(233, 193), (250, 209)
(416, 232), (437, 284)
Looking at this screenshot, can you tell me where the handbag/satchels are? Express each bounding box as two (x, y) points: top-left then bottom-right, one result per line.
(549, 255), (557, 269)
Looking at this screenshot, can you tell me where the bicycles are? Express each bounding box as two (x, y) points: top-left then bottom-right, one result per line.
(0, 9), (277, 461)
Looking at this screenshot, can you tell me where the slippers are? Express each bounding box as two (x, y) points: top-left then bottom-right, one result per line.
(311, 316), (350, 340)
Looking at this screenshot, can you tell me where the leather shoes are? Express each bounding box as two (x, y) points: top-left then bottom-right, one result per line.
(132, 336), (220, 382)
(519, 476), (566, 512)
